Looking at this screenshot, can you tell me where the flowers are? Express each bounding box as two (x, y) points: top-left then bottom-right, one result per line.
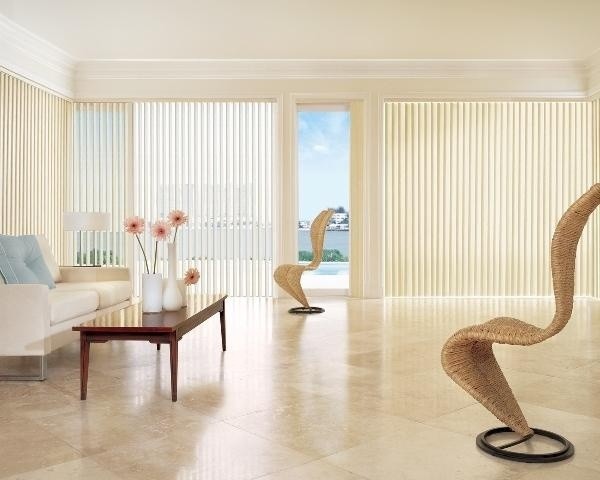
(115, 206), (171, 275)
(166, 209), (188, 244)
(180, 266), (202, 287)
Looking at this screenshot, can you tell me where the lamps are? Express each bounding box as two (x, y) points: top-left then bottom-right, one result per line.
(64, 209), (111, 268)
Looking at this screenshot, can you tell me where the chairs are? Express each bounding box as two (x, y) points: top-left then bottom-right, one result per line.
(435, 181), (600, 464)
(271, 207), (335, 316)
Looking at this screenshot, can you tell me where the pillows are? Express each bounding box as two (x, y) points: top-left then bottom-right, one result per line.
(0, 232), (56, 288)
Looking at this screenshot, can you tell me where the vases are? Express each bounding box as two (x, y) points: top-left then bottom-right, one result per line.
(141, 273), (163, 314)
(164, 241), (179, 281)
(161, 282), (183, 312)
(178, 282), (189, 307)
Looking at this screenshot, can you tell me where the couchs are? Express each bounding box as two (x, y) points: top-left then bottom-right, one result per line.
(0, 236), (135, 382)
